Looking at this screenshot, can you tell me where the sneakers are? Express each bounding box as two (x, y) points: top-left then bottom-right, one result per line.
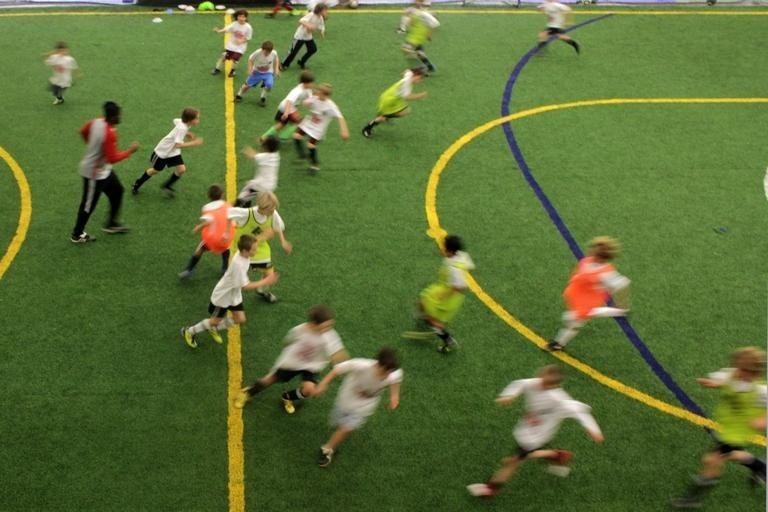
(234, 388), (250, 409)
(280, 394), (294, 413)
(69, 232), (95, 244)
(467, 482), (498, 498)
(437, 340), (458, 353)
(203, 322), (223, 344)
(101, 224), (129, 234)
(181, 326), (199, 350)
(53, 99), (64, 105)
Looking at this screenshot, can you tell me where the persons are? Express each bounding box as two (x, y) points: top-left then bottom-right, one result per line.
(672, 348), (767, 509)
(235, 305), (348, 414)
(548, 236), (634, 351)
(416, 235), (476, 352)
(179, 185), (292, 348)
(211, 1), (351, 175)
(234, 134), (282, 207)
(363, 65), (428, 136)
(536, 1), (581, 54)
(131, 107), (204, 198)
(70, 101), (140, 244)
(465, 365), (605, 499)
(44, 42), (77, 105)
(313, 347), (405, 467)
(397, 1), (441, 75)
